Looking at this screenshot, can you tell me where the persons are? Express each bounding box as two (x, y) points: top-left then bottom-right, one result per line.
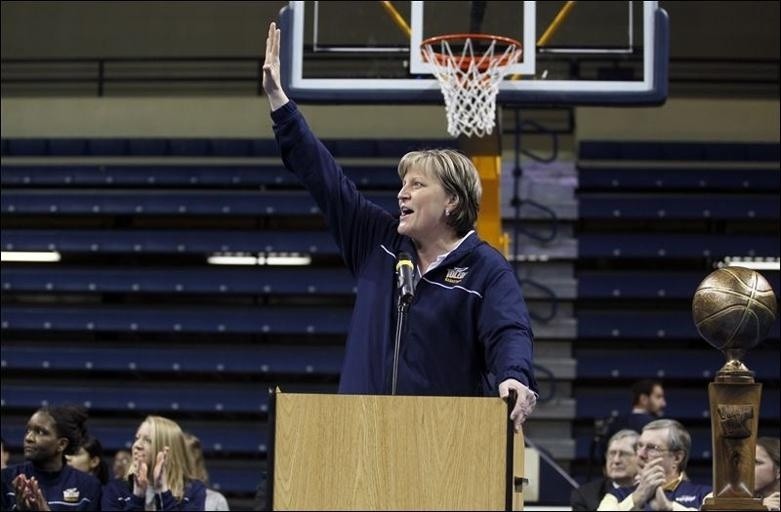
(256, 16), (538, 434)
(569, 379), (781, 512)
(0, 400), (233, 511)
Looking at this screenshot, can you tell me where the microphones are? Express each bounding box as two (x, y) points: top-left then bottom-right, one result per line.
(395, 251), (416, 305)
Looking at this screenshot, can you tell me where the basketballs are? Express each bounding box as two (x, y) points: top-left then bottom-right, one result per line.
(692, 267), (777, 350)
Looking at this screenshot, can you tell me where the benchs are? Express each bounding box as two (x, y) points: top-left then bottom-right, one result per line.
(1, 136), (781, 500)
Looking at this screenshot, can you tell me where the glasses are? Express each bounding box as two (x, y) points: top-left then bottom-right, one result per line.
(637, 441), (681, 454)
